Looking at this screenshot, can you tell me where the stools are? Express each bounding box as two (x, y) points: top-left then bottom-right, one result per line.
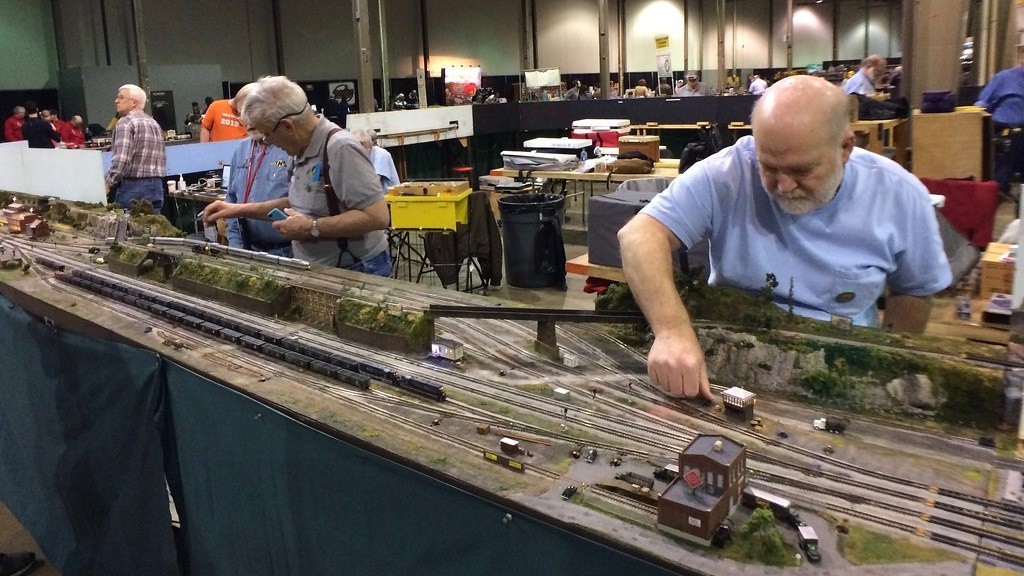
(451, 166), (474, 180)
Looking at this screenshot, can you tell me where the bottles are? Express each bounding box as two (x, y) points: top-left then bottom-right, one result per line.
(580, 148), (587, 162)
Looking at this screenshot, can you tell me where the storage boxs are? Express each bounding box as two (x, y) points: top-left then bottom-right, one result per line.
(982, 292), (1013, 326)
(847, 94), (858, 123)
(523, 119), (660, 161)
(980, 241), (1015, 299)
(383, 181), (472, 232)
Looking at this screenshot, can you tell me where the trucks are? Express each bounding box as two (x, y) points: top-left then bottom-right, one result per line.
(740, 486), (805, 529)
(798, 523), (822, 563)
(813, 415), (851, 435)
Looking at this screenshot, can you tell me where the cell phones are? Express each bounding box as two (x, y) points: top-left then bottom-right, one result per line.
(267, 208), (289, 222)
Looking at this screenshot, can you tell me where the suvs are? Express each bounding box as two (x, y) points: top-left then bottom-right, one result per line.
(653, 464), (679, 482)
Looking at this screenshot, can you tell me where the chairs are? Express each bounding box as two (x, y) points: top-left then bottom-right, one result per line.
(920, 178), (998, 251)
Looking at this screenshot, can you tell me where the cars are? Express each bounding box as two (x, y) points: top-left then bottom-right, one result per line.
(561, 485), (578, 500)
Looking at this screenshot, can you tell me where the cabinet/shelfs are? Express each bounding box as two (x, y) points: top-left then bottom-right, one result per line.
(912, 107), (994, 182)
(851, 116), (911, 167)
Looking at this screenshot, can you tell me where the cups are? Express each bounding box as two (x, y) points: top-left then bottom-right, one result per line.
(167, 180), (176, 193)
(594, 159), (606, 173)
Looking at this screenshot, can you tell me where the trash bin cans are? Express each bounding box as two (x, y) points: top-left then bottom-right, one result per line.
(497, 193), (566, 289)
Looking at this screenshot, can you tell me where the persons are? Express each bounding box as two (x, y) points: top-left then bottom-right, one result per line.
(21, 100), (65, 149)
(41, 110), (58, 147)
(201, 75), (392, 277)
(106, 84), (166, 215)
(394, 89), (418, 104)
(617, 75), (952, 402)
(842, 55), (887, 97)
(4, 105), (26, 142)
(565, 65), (902, 101)
(365, 128), (379, 146)
(325, 95), (350, 121)
(62, 115), (85, 145)
(349, 128), (401, 195)
(185, 96), (214, 125)
(975, 44), (1024, 196)
(374, 98), (378, 110)
(472, 87), (500, 104)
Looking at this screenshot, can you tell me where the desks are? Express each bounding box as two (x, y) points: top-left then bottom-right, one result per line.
(629, 124), (711, 135)
(574, 145), (681, 200)
(490, 168), (680, 224)
(168, 186), (228, 234)
(384, 225), (473, 295)
(564, 253), (627, 285)
(728, 124), (752, 144)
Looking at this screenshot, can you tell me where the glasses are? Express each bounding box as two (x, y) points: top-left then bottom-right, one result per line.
(116, 95), (136, 100)
(263, 115), (286, 146)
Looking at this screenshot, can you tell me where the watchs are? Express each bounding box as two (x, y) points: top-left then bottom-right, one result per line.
(311, 217), (320, 240)
